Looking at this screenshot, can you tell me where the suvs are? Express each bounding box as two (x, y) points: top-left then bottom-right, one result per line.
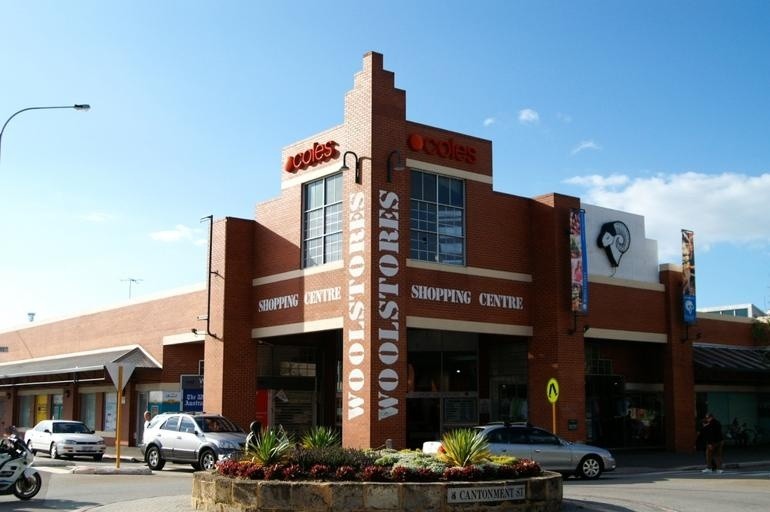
(140, 412), (248, 471)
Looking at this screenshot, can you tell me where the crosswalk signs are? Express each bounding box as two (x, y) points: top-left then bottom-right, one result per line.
(547, 377), (559, 402)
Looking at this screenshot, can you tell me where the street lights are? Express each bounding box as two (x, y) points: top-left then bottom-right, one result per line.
(0, 105), (90, 147)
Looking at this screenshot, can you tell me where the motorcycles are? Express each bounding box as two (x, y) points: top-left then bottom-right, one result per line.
(0, 435), (40, 500)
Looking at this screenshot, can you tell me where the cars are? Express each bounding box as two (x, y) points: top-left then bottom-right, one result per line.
(473, 422), (616, 480)
(24, 420), (106, 460)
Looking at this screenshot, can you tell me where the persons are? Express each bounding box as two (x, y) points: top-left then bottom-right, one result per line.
(144, 411), (152, 429)
(208, 420), (220, 432)
(245, 421), (262, 455)
(702, 413), (748, 473)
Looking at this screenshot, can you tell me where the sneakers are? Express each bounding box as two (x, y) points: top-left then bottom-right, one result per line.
(702, 468), (712, 472)
(717, 469), (723, 473)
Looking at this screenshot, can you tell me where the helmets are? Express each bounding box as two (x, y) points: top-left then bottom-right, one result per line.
(250, 421), (262, 433)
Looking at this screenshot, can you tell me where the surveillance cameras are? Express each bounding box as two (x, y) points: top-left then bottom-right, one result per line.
(196, 314), (208, 320)
